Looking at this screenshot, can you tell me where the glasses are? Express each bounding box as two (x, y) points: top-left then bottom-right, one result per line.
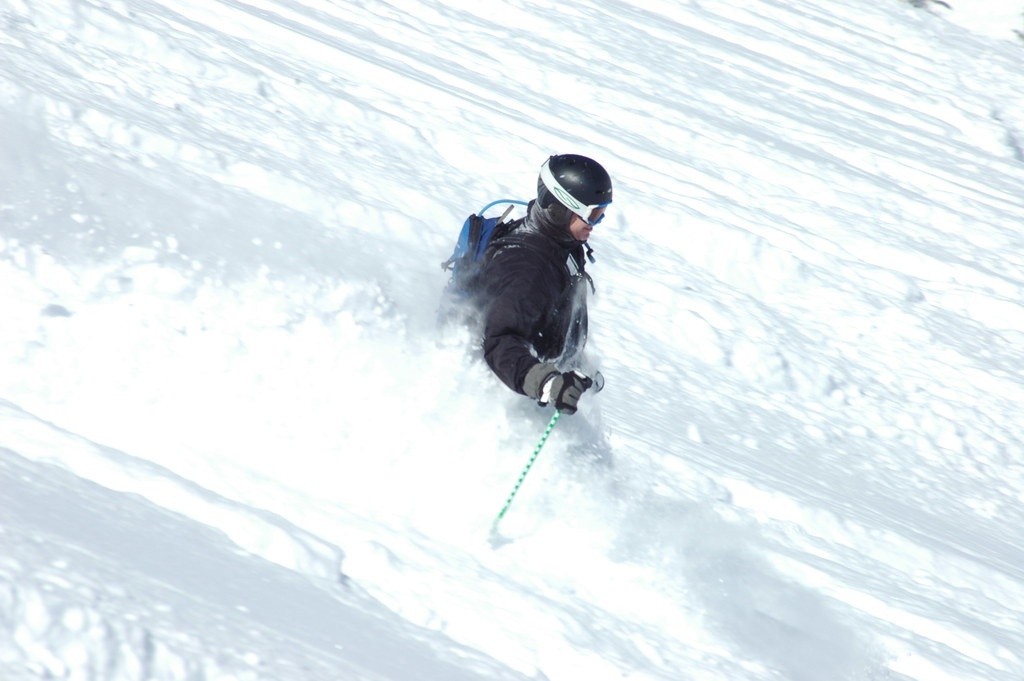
(582, 202), (613, 225)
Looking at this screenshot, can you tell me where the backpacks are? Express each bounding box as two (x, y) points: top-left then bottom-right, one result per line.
(442, 213), (572, 304)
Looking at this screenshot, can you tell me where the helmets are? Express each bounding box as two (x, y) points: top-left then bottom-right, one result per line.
(537, 154), (613, 227)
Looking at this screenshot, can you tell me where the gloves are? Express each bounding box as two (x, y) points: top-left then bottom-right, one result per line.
(521, 362), (592, 415)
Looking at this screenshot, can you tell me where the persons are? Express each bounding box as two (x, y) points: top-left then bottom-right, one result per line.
(479, 150), (614, 413)
